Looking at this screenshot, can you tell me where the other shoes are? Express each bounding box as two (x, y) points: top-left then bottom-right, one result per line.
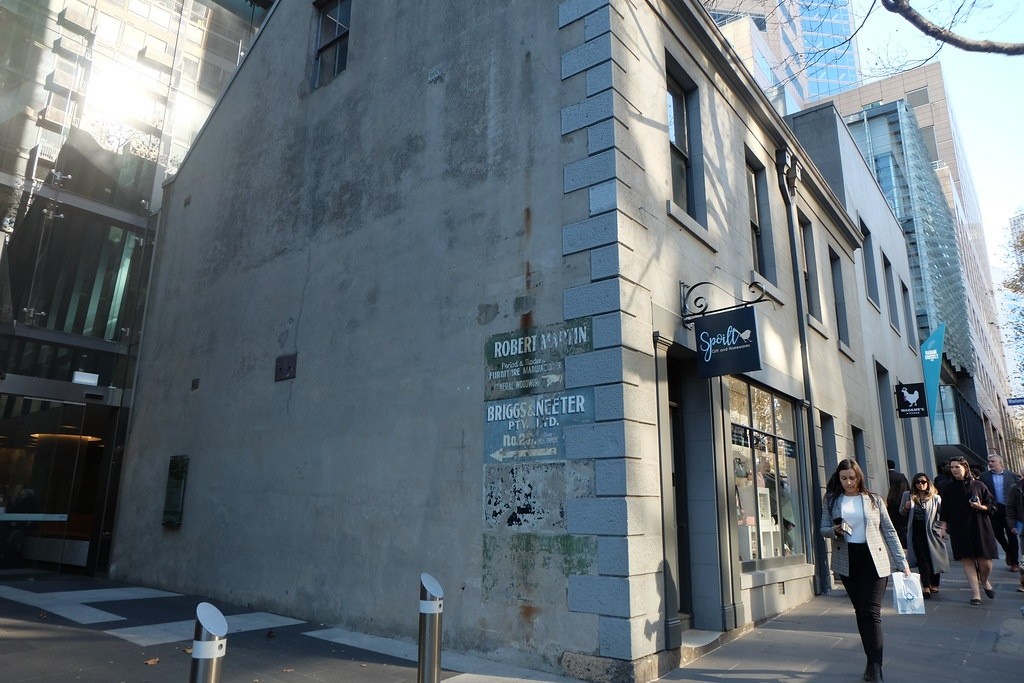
(1009, 564), (1021, 572)
(1017, 585), (1024, 592)
(984, 586), (995, 599)
(864, 656), (884, 683)
(970, 598), (982, 605)
(929, 585), (939, 593)
(923, 586), (931, 598)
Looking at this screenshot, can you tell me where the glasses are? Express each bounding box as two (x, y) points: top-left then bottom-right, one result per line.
(914, 479), (927, 485)
(949, 456), (964, 461)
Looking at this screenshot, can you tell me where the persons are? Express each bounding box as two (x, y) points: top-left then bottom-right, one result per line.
(1005, 469), (1024, 592)
(939, 457), (998, 604)
(820, 460), (910, 683)
(979, 455), (1020, 571)
(887, 459), (910, 492)
(899, 473), (942, 597)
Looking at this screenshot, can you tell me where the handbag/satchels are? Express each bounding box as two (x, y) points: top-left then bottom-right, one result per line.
(892, 572), (926, 615)
(898, 521), (907, 549)
